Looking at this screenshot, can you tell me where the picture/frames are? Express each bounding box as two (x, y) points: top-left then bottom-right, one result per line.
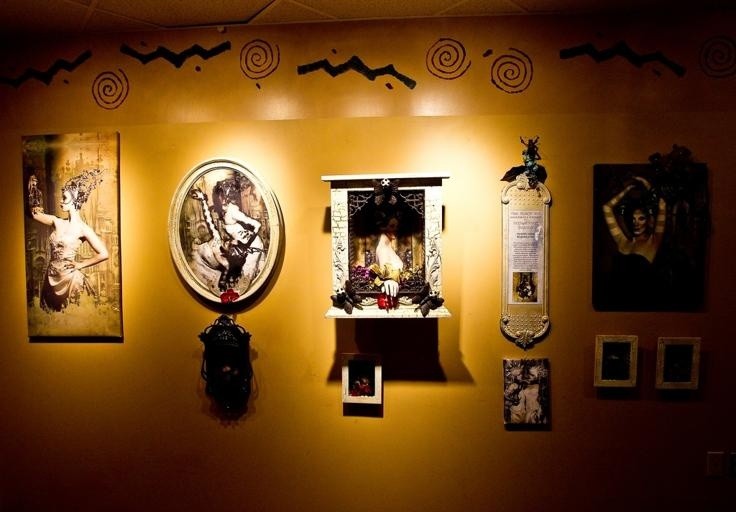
(166, 156), (284, 310)
(590, 331), (643, 391)
(653, 332), (705, 395)
(338, 349), (386, 409)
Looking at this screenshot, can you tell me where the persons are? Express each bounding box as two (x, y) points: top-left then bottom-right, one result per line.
(189, 176), (266, 296)
(602, 174), (668, 307)
(504, 366), (546, 423)
(375, 217), (404, 271)
(27, 169), (110, 312)
